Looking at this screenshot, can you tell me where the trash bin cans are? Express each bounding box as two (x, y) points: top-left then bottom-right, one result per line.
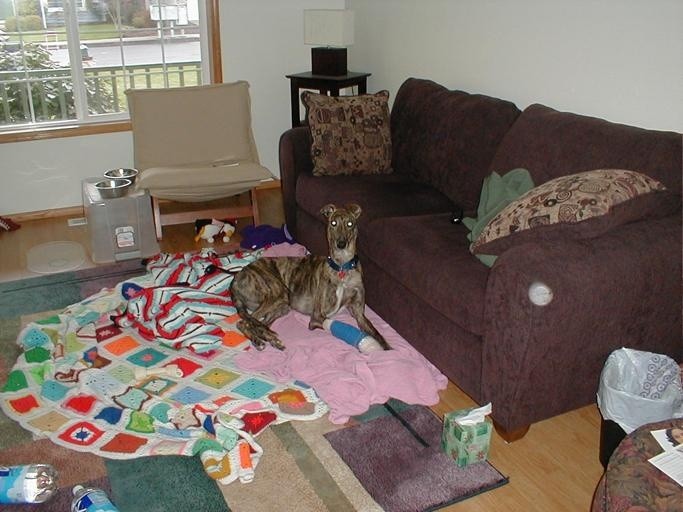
(596, 345), (683, 471)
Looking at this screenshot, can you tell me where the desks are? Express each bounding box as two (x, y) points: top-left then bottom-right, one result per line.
(286, 73), (371, 128)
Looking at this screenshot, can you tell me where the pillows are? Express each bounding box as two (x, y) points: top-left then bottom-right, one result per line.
(301, 91), (394, 178)
(470, 168), (683, 252)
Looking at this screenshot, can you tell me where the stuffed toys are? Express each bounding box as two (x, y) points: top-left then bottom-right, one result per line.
(191, 216), (238, 244)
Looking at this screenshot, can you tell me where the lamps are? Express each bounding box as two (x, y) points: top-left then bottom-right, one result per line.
(302, 8), (356, 76)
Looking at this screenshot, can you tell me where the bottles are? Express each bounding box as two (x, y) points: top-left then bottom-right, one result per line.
(0, 463), (59, 506)
(69, 485), (120, 511)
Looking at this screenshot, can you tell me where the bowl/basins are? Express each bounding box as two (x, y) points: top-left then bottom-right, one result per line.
(92, 179), (132, 198)
(104, 167), (139, 184)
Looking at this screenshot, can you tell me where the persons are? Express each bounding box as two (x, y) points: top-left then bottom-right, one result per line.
(666, 426), (683, 449)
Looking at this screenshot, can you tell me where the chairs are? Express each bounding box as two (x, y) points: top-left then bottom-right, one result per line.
(125, 81), (277, 241)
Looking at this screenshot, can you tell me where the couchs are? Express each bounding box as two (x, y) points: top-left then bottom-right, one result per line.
(591, 415), (683, 512)
(279, 77), (682, 442)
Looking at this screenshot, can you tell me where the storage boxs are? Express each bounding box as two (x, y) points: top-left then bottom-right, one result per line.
(82, 176), (161, 263)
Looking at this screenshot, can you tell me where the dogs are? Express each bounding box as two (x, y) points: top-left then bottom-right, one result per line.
(211, 202), (394, 352)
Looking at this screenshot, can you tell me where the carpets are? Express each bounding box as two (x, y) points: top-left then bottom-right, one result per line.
(0, 248), (509, 511)
